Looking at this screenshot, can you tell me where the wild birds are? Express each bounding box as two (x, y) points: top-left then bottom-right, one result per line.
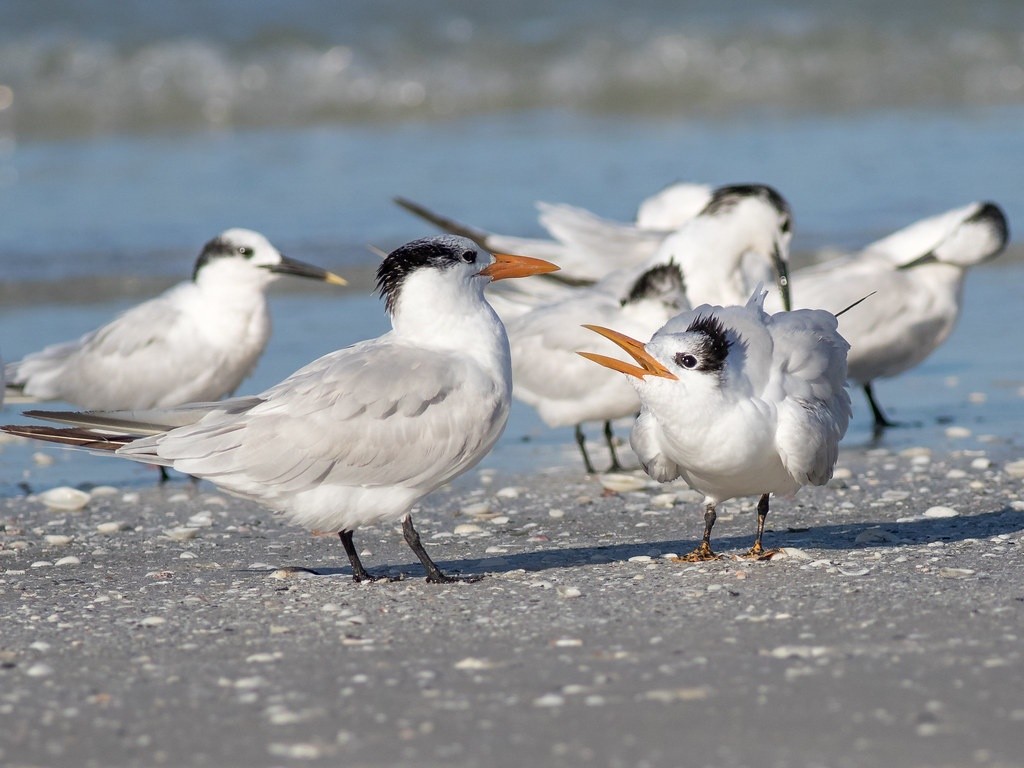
(0, 180), (1009, 584)
